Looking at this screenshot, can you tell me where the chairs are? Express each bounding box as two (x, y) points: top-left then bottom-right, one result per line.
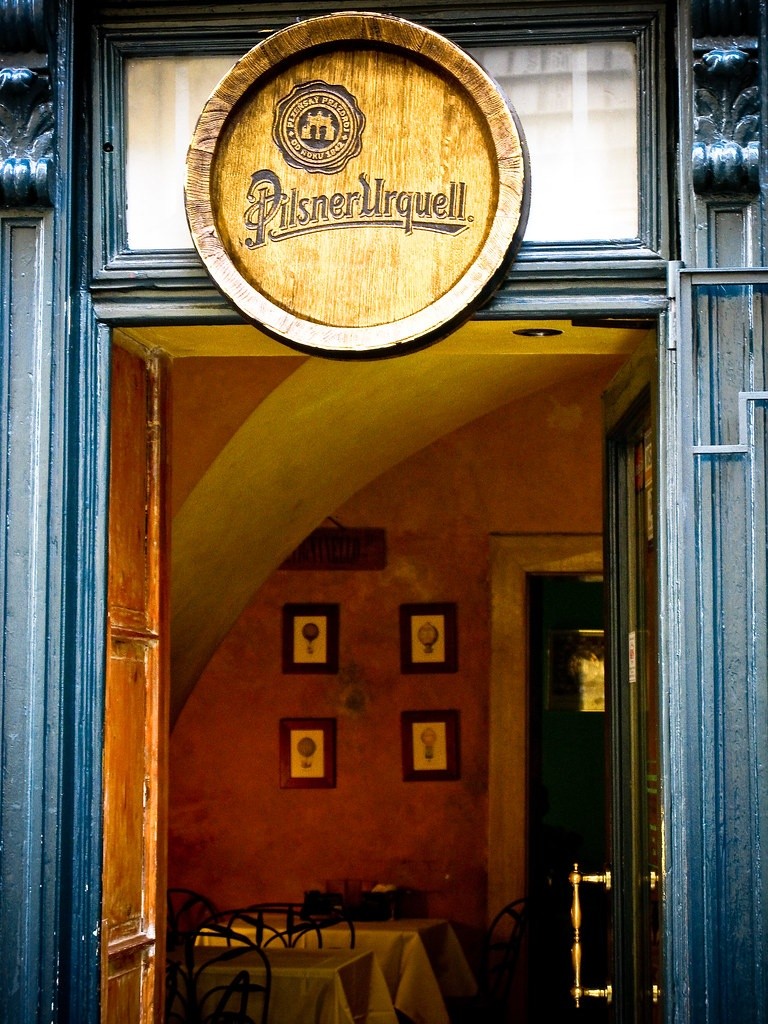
(166, 883), (357, 1023)
(466, 897), (533, 1024)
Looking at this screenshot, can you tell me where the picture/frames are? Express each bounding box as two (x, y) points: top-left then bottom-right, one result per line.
(399, 710), (460, 780)
(542, 628), (605, 713)
(278, 718), (336, 787)
(283, 603), (337, 674)
(399, 603), (458, 676)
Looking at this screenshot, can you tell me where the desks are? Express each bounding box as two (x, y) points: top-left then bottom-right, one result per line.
(167, 944), (397, 1024)
(196, 910), (476, 1024)
